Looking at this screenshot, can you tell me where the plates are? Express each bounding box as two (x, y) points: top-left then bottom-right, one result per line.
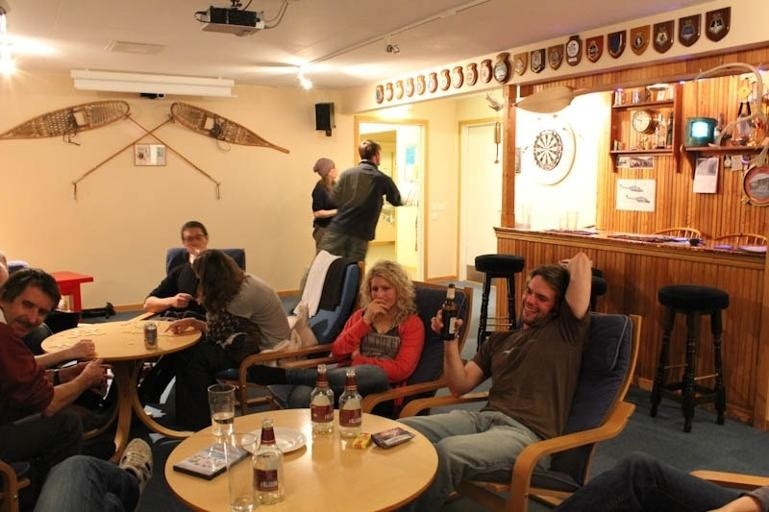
(242, 424), (310, 455)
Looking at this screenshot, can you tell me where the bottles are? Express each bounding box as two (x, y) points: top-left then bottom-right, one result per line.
(651, 110), (672, 149)
(713, 112), (726, 145)
(441, 283), (459, 342)
(338, 366), (364, 437)
(309, 362), (335, 434)
(254, 416), (287, 504)
(613, 87), (624, 106)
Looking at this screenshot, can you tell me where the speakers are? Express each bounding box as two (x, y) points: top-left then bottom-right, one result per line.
(315, 102), (334, 131)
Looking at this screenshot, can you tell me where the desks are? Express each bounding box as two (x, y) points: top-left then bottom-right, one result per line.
(40, 320), (204, 466)
(163, 406), (442, 512)
(49, 270), (94, 322)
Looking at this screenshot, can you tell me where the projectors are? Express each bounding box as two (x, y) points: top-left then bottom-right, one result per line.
(199, 6), (264, 35)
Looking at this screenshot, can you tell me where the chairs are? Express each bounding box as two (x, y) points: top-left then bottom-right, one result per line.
(713, 228), (766, 248)
(279, 281), (475, 417)
(650, 226), (704, 246)
(126, 247), (246, 323)
(398, 310), (644, 511)
(690, 468), (769, 490)
(1, 459), (33, 512)
(217, 251), (367, 415)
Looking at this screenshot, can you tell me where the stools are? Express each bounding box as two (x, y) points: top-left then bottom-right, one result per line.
(474, 254), (526, 352)
(645, 284), (730, 433)
(589, 273), (606, 312)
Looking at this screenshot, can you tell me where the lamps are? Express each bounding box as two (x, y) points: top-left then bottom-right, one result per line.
(383, 33), (401, 56)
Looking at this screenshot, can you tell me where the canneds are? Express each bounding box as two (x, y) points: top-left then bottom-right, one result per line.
(144, 324), (157, 349)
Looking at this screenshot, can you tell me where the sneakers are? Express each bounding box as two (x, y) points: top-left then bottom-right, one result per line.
(118, 438), (154, 512)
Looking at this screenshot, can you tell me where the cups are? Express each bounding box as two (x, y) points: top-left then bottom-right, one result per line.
(52, 321), (106, 349)
(207, 383), (236, 434)
(222, 432), (256, 511)
(120, 311), (177, 335)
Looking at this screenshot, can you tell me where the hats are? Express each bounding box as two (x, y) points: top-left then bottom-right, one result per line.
(313, 157), (335, 178)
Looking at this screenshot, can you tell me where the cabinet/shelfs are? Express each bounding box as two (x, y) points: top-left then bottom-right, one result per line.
(608, 82), (683, 173)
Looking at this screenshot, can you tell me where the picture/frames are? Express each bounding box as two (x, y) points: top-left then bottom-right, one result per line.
(132, 142), (167, 167)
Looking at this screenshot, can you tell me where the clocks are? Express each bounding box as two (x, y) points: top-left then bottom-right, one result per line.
(631, 109), (653, 135)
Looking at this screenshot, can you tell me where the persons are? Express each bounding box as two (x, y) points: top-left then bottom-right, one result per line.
(310, 154), (339, 256)
(34, 437), (155, 511)
(550, 451), (768, 512)
(287, 140), (406, 312)
(135, 221), (216, 405)
(0, 267), (106, 461)
(135, 249), (291, 410)
(247, 260), (425, 414)
(0, 252), (53, 358)
(394, 251), (592, 512)
